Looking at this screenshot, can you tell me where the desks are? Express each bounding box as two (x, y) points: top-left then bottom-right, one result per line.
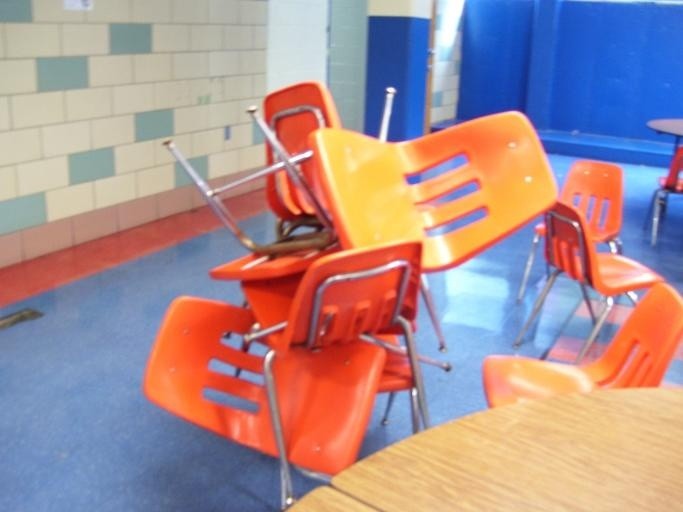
(649, 119), (681, 137)
(288, 386), (682, 510)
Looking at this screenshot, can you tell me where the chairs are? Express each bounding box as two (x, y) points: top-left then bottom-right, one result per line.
(482, 156), (682, 408)
(649, 146), (683, 247)
(142, 79), (560, 509)
